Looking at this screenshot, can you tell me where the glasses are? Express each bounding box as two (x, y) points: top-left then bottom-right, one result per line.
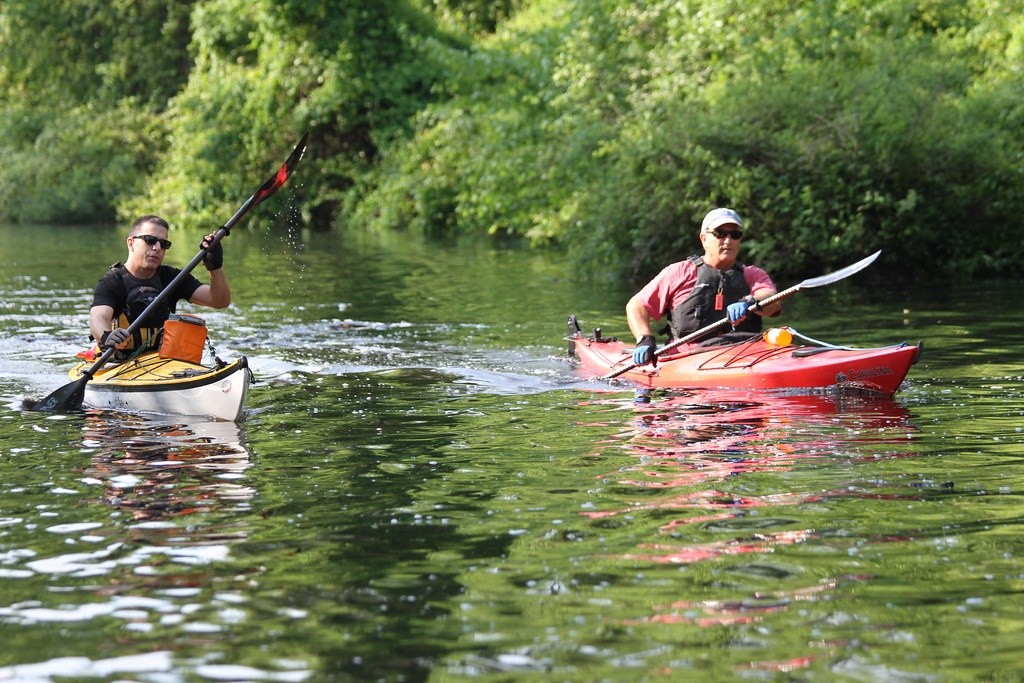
(705, 229), (743, 240)
(133, 235), (171, 249)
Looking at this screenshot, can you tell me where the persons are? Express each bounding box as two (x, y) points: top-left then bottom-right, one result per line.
(89, 215), (230, 366)
(626, 207), (781, 369)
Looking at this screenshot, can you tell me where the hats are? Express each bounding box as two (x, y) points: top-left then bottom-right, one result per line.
(701, 208), (742, 233)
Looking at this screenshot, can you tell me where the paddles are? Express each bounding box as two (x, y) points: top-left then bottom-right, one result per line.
(28, 127), (313, 415)
(597, 247), (884, 382)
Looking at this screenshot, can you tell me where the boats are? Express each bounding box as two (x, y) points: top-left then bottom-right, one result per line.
(69, 346), (251, 422)
(561, 314), (923, 397)
